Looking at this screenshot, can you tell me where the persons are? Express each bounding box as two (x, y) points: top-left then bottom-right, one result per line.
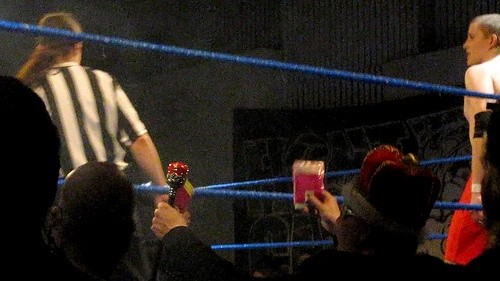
(242, 248), (316, 280)
(150, 147), (472, 281)
(460, 130), (500, 281)
(50, 161), (145, 281)
(443, 14), (500, 266)
(18, 12), (173, 281)
(0, 76), (62, 281)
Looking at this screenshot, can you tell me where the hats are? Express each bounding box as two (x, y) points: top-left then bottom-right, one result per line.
(341, 145), (443, 239)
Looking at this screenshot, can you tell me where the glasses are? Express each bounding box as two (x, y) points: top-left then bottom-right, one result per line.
(340, 203), (359, 223)
